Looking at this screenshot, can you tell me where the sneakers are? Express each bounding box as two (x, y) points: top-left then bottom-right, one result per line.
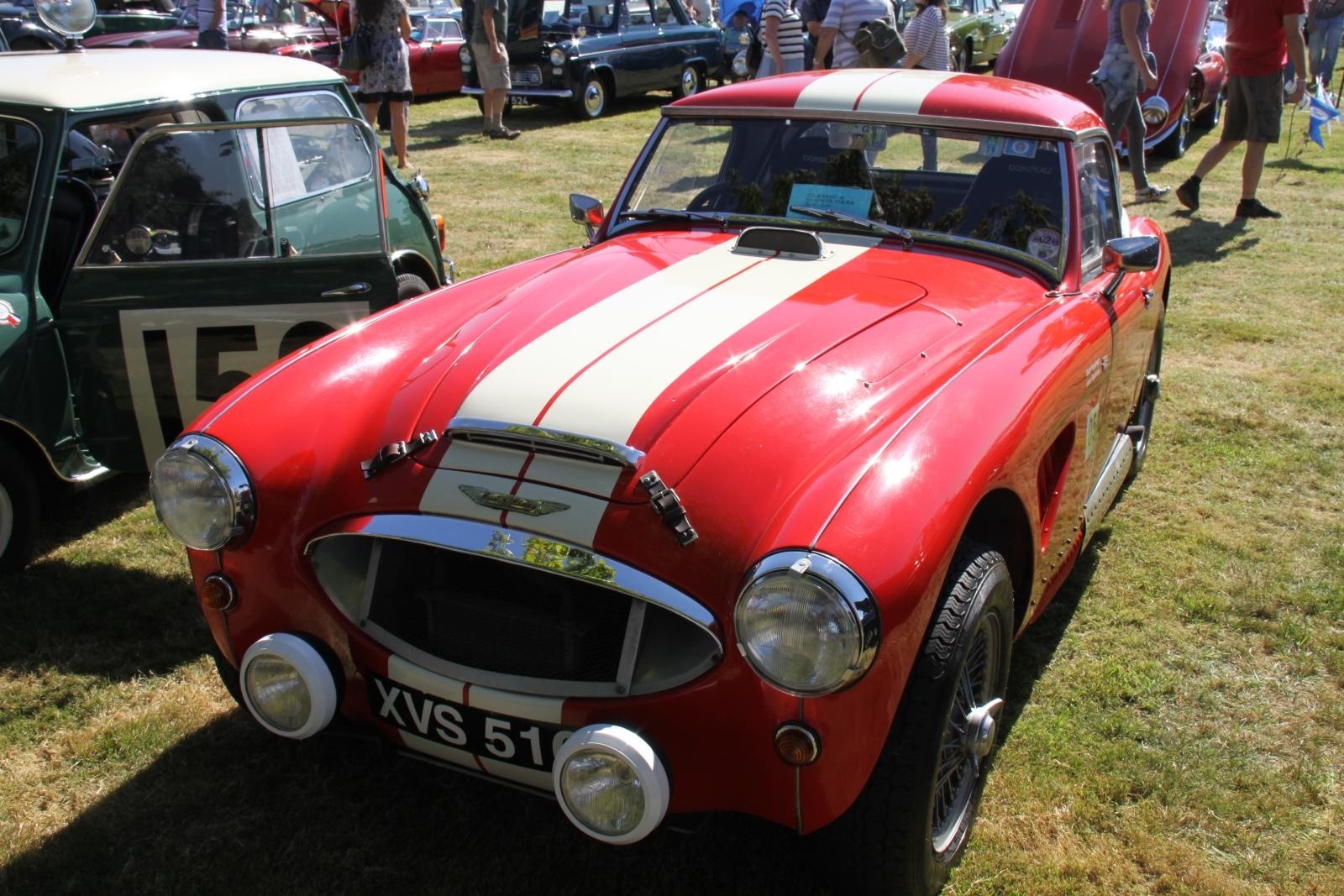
(1134, 186), (1170, 203)
(1177, 181), (1200, 210)
(1236, 202), (1279, 218)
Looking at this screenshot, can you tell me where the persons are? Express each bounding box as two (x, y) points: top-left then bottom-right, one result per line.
(755, 0), (896, 167)
(897, 0), (950, 171)
(681, 0), (752, 87)
(1174, 0), (1308, 218)
(259, 0), (309, 27)
(1086, 0), (1171, 202)
(197, 0), (229, 50)
(1283, 0), (1344, 104)
(350, 0), (412, 168)
(469, 0), (521, 139)
(92, 40), (184, 140)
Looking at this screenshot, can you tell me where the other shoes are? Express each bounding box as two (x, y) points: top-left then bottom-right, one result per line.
(398, 164), (413, 169)
(483, 126), (521, 140)
(1305, 82), (1318, 93)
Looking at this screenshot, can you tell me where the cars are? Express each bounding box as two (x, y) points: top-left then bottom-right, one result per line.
(0, 1), (468, 108)
(458, 0), (725, 122)
(989, 0), (1230, 156)
(0, 52), (461, 592)
(892, 1), (1022, 77)
(149, 66), (1170, 895)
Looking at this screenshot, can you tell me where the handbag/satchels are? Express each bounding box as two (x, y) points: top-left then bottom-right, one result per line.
(340, 25), (361, 71)
(745, 26), (763, 69)
(854, 21), (908, 67)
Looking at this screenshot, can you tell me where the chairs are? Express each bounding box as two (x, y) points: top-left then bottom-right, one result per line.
(952, 149), (1088, 269)
(753, 136), (886, 223)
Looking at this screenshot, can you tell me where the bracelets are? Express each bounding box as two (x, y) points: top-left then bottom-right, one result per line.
(813, 59), (820, 65)
